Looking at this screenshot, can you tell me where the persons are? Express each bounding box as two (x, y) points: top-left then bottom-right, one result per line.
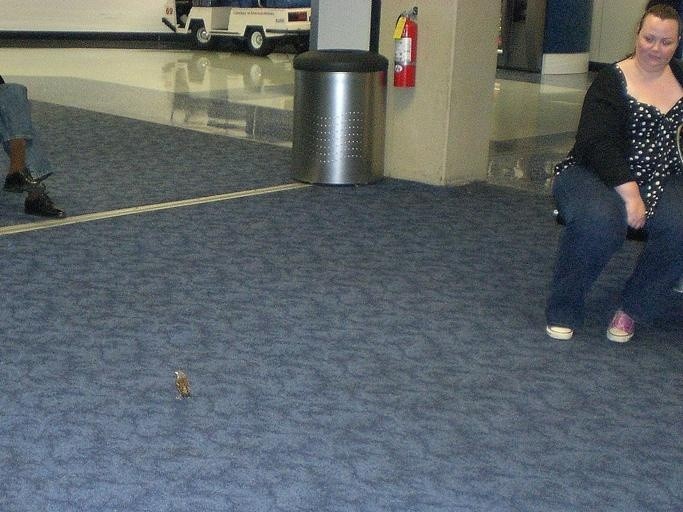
(0, 72), (68, 218)
(543, 3), (683, 344)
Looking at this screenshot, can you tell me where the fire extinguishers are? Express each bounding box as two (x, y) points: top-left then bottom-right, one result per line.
(393, 6), (419, 87)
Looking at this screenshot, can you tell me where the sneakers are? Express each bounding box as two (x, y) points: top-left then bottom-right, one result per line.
(546, 322), (575, 340)
(607, 307), (638, 343)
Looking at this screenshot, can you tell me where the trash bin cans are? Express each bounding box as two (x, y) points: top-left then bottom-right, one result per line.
(294, 49), (388, 186)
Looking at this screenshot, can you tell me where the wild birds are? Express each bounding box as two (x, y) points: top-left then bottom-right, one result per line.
(173, 368), (193, 401)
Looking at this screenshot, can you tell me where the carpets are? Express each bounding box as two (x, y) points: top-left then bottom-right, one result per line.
(0, 99), (682, 512)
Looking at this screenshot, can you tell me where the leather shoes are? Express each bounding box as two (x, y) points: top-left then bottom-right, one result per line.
(3, 167), (66, 219)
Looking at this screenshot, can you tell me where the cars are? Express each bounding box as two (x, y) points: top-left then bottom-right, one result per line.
(160, 0), (311, 56)
(161, 56), (303, 143)
